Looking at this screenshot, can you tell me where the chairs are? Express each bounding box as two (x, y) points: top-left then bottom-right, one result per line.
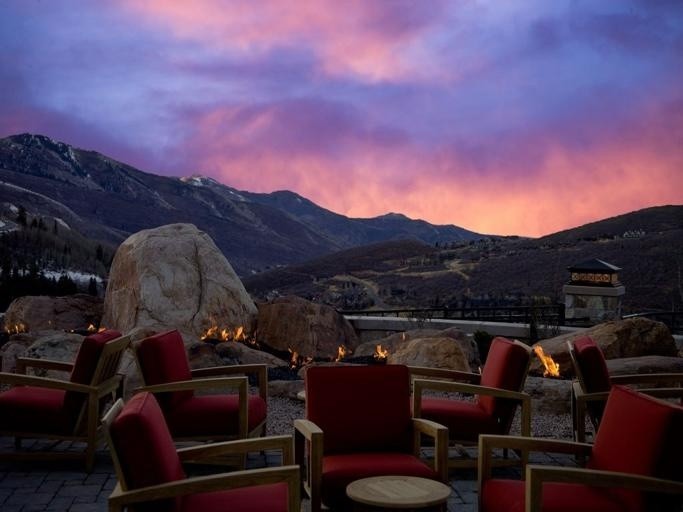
(292, 361), (453, 510)
(100, 389), (304, 511)
(406, 334), (537, 481)
(565, 333), (681, 458)
(131, 330), (270, 472)
(473, 385), (682, 512)
(0, 330), (130, 469)
